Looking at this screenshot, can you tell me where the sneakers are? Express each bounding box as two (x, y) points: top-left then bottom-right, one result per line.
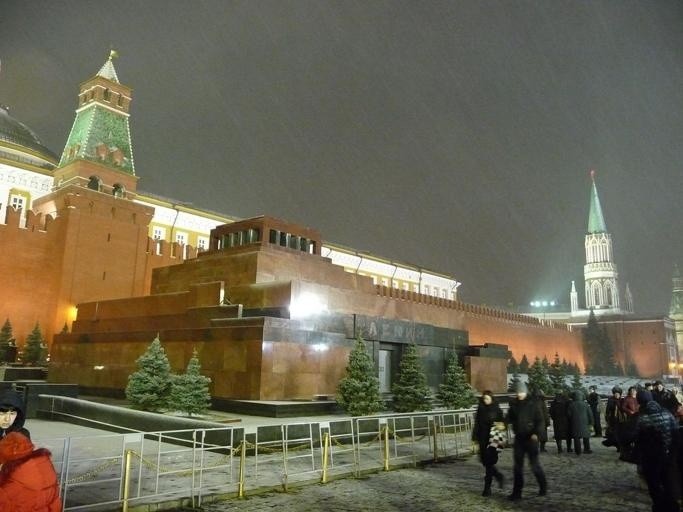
(558, 448), (593, 454)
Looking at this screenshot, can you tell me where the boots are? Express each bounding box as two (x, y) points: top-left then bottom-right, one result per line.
(482, 476), (492, 496)
(494, 469), (504, 488)
(507, 488), (522, 500)
(538, 480), (547, 495)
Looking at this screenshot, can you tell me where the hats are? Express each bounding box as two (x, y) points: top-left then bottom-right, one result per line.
(0, 432), (35, 464)
(516, 383), (527, 393)
(612, 387), (622, 394)
(637, 390), (653, 404)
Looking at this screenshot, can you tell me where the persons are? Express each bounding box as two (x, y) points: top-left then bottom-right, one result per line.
(469, 388), (505, 497)
(505, 380), (547, 502)
(0, 430), (63, 512)
(0, 387), (31, 441)
(532, 378), (682, 511)
